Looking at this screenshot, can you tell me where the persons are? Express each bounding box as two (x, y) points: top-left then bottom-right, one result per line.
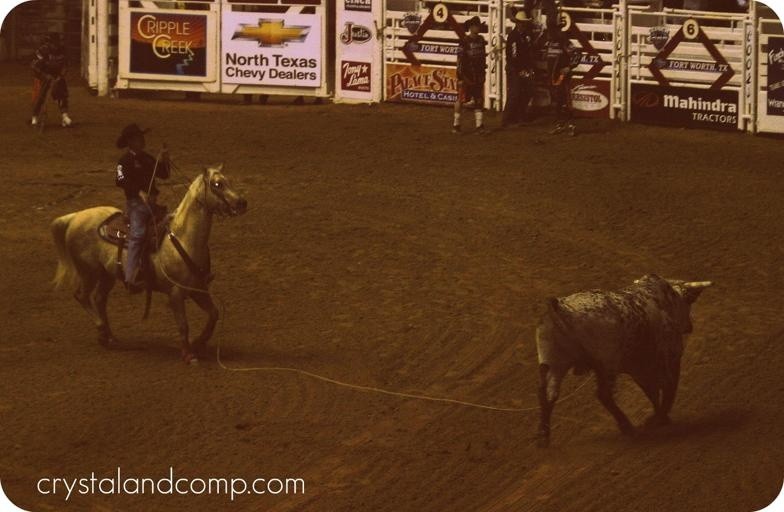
(30, 32), (72, 127)
(452, 11), (537, 135)
(116, 125), (170, 288)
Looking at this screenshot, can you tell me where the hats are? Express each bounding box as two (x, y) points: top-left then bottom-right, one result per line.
(464, 16), (486, 28)
(510, 11), (532, 24)
(114, 121), (151, 149)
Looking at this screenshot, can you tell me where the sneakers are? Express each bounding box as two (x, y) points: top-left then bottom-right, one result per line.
(451, 119), (577, 138)
(30, 114), (73, 130)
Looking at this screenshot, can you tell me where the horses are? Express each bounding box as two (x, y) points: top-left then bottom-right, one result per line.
(51, 162), (249, 368)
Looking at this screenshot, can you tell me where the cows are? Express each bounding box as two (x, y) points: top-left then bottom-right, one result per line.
(534, 272), (715, 448)
